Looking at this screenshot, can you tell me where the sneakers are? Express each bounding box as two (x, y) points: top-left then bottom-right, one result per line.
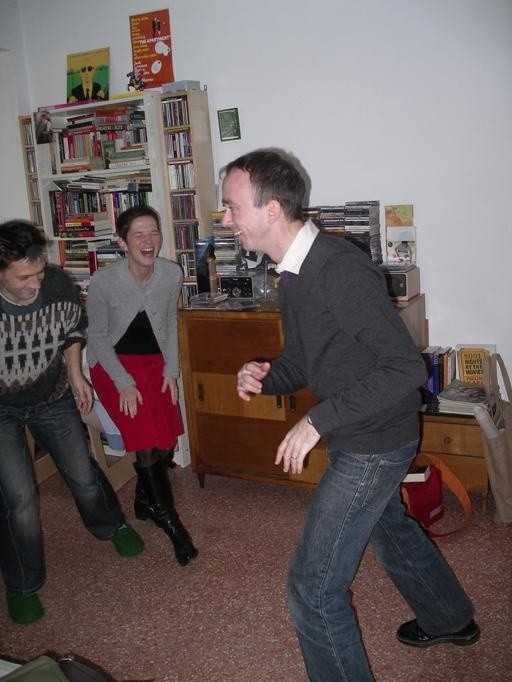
(111, 522), (145, 557)
(5, 589), (44, 625)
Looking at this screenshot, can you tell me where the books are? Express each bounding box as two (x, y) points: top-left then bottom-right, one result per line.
(24, 81), (228, 310)
(421, 345), (498, 415)
(402, 465), (431, 482)
(211, 201), (382, 276)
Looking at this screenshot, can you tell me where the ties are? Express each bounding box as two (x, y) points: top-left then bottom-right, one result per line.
(85, 88), (90, 100)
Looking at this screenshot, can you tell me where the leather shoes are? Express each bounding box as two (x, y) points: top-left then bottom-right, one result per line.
(396, 618), (481, 648)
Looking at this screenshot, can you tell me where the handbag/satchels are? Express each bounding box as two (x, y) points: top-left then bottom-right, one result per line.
(399, 451), (472, 538)
(480, 352), (512, 524)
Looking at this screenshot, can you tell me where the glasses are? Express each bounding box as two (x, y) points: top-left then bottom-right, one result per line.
(80, 65), (93, 72)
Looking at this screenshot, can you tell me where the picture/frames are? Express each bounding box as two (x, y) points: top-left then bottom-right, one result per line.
(217, 108), (241, 141)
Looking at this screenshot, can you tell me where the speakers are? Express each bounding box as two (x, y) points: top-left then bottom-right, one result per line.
(383, 267), (421, 302)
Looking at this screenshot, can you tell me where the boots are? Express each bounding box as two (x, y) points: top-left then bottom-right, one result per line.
(132, 450), (199, 568)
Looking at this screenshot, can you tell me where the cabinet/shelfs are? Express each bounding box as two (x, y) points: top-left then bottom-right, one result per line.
(178, 294), (429, 492)
(18, 90), (217, 469)
(408, 404), (504, 515)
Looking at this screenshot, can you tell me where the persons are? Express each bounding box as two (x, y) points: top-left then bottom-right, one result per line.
(85, 204), (198, 567)
(221, 148), (480, 682)
(68, 60), (105, 104)
(2, 206), (146, 625)
